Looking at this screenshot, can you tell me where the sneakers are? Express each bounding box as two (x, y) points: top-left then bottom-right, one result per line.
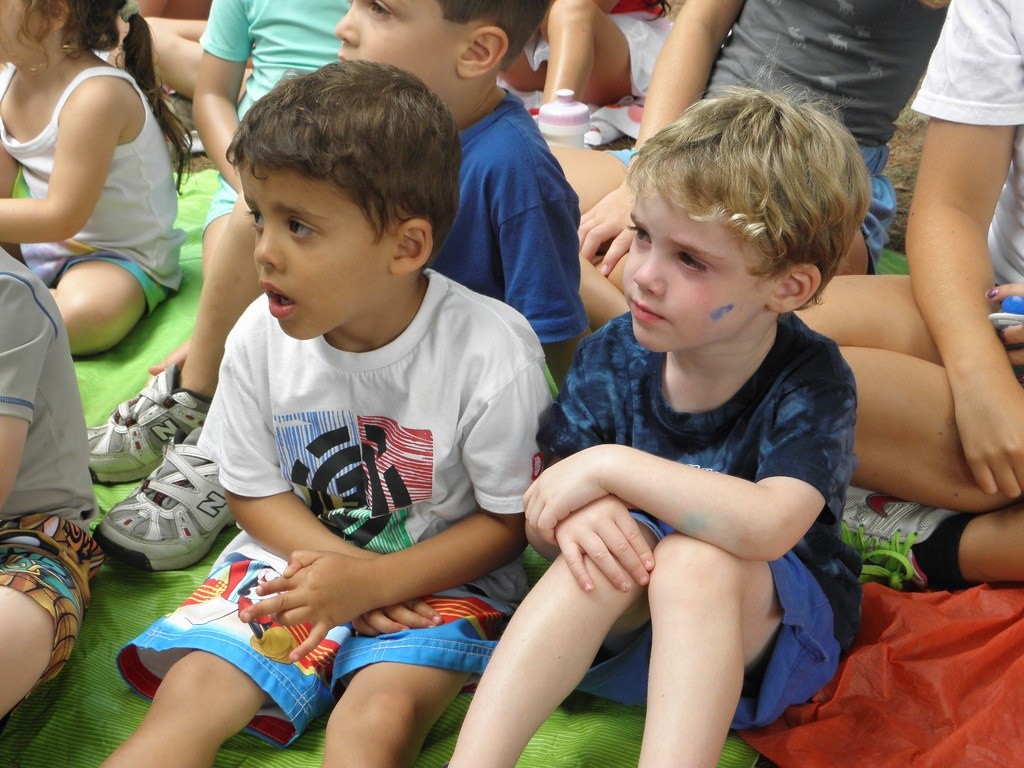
(92, 424), (236, 572)
(838, 485), (964, 593)
(86, 363), (213, 485)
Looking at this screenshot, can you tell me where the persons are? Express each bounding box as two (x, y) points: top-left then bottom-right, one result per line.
(447, 88), (872, 768)
(101, 61), (556, 768)
(86, 0), (1024, 591)
(0, 245), (99, 723)
(0, 1), (192, 358)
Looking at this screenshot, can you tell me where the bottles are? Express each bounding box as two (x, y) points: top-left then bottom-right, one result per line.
(537, 89), (592, 150)
